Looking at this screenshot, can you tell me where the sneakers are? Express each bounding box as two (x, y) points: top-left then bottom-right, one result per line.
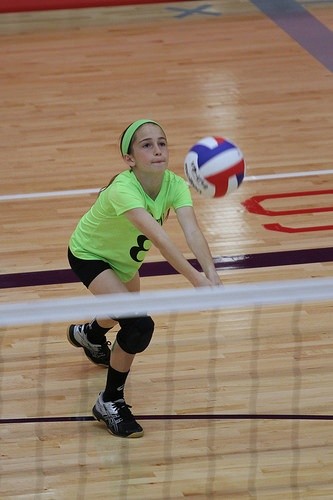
(67, 323), (112, 368)
(92, 391), (144, 437)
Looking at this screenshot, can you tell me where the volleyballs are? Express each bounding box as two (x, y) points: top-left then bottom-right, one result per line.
(184, 136), (245, 198)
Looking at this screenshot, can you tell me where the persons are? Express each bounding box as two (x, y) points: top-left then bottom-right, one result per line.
(66, 119), (224, 438)
